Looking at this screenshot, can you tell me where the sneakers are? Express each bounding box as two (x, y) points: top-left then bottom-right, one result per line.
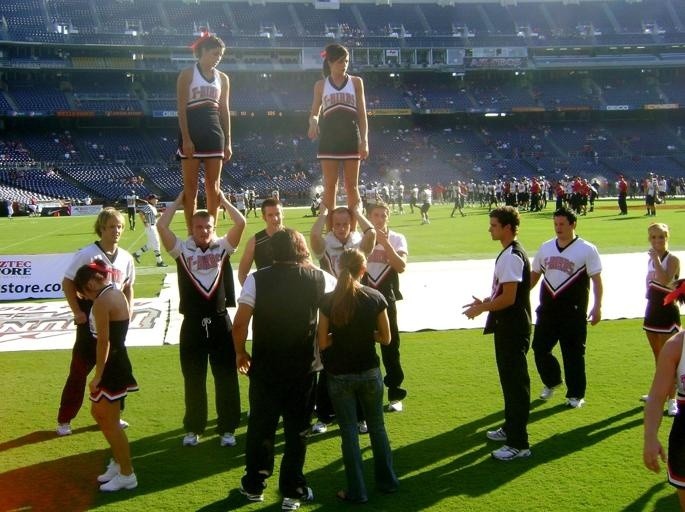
(488, 427), (530, 459)
(540, 380), (561, 400)
(120, 419), (129, 429)
(360, 418), (369, 433)
(57, 423), (73, 436)
(337, 490), (367, 504)
(325, 232), (342, 248)
(566, 396), (582, 408)
(668, 398), (677, 417)
(97, 460), (137, 491)
(183, 433), (199, 446)
(280, 487), (314, 510)
(388, 400), (402, 412)
(240, 483), (264, 501)
(345, 230), (363, 249)
(313, 420), (333, 432)
(187, 235), (197, 250)
(220, 433), (236, 447)
(209, 234), (219, 249)
(641, 394), (647, 402)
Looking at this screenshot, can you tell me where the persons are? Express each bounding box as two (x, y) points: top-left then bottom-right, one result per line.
(461, 203), (530, 462)
(642, 222), (681, 416)
(129, 192), (169, 267)
(372, 45), (685, 226)
(57, 207), (135, 437)
(231, 2), (308, 210)
(307, 44), (369, 237)
(308, 5), (685, 44)
(643, 277), (685, 512)
(241, 195), (410, 512)
(530, 208), (603, 409)
(2, 5), (179, 252)
(157, 181), (246, 448)
(71, 255), (142, 494)
(176, 31), (232, 244)
